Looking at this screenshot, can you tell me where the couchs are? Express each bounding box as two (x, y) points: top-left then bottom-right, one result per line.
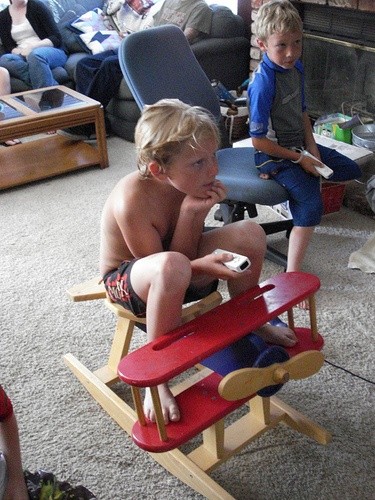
(57, 0), (250, 142)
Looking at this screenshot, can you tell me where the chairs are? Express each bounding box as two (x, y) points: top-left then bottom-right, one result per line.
(118, 24), (293, 273)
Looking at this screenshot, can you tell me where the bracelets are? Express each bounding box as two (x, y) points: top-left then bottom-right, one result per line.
(291, 147), (302, 163)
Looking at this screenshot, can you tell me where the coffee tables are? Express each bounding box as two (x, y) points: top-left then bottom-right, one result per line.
(0, 85), (109, 190)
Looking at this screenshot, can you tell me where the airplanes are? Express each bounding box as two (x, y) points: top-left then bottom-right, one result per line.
(56, 253), (333, 499)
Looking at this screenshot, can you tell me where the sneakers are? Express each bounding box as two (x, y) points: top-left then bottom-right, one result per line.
(56, 125), (92, 141)
(90, 129), (110, 139)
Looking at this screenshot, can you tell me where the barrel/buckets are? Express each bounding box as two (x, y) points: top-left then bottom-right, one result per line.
(351, 124), (375, 186)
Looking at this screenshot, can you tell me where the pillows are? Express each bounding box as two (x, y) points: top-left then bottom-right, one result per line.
(66, 6), (114, 34)
(74, 31), (121, 56)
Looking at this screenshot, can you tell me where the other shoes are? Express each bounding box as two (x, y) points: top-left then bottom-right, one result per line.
(0, 138), (22, 147)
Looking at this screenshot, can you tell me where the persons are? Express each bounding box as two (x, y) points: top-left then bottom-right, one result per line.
(0, 1), (67, 135)
(246, 1), (363, 312)
(56, 0), (213, 142)
(98, 98), (298, 426)
(0, 66), (22, 147)
